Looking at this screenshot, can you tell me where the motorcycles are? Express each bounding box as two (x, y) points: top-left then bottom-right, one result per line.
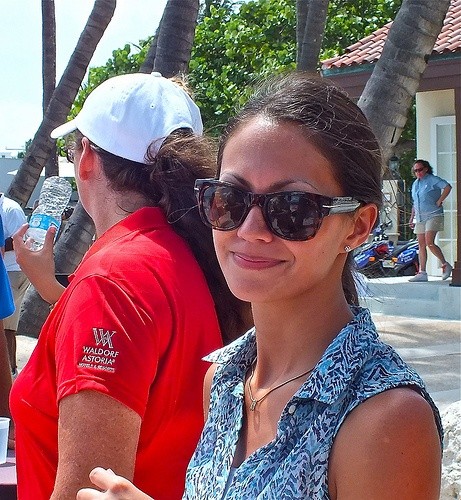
(351, 220), (421, 279)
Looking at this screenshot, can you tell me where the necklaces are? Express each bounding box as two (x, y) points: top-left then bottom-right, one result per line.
(248, 355), (315, 412)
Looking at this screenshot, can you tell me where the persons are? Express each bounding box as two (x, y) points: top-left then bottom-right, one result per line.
(211, 190), (244, 228)
(9, 70), (246, 500)
(76, 70), (444, 500)
(0, 193), (31, 378)
(0, 214), (15, 419)
(12, 222), (65, 307)
(408, 160), (453, 281)
(273, 195), (318, 238)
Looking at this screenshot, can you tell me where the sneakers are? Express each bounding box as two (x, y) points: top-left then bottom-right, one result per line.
(408, 273), (428, 282)
(442, 262), (452, 280)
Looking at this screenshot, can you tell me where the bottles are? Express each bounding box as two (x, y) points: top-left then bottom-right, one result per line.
(22, 176), (72, 252)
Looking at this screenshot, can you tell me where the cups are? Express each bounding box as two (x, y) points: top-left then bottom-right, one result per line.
(0, 417), (11, 465)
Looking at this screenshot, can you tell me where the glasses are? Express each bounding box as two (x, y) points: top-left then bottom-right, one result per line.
(414, 167), (425, 172)
(194, 179), (360, 241)
(63, 143), (101, 164)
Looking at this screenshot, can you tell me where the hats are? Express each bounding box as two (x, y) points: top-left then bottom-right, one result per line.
(51, 72), (203, 165)
